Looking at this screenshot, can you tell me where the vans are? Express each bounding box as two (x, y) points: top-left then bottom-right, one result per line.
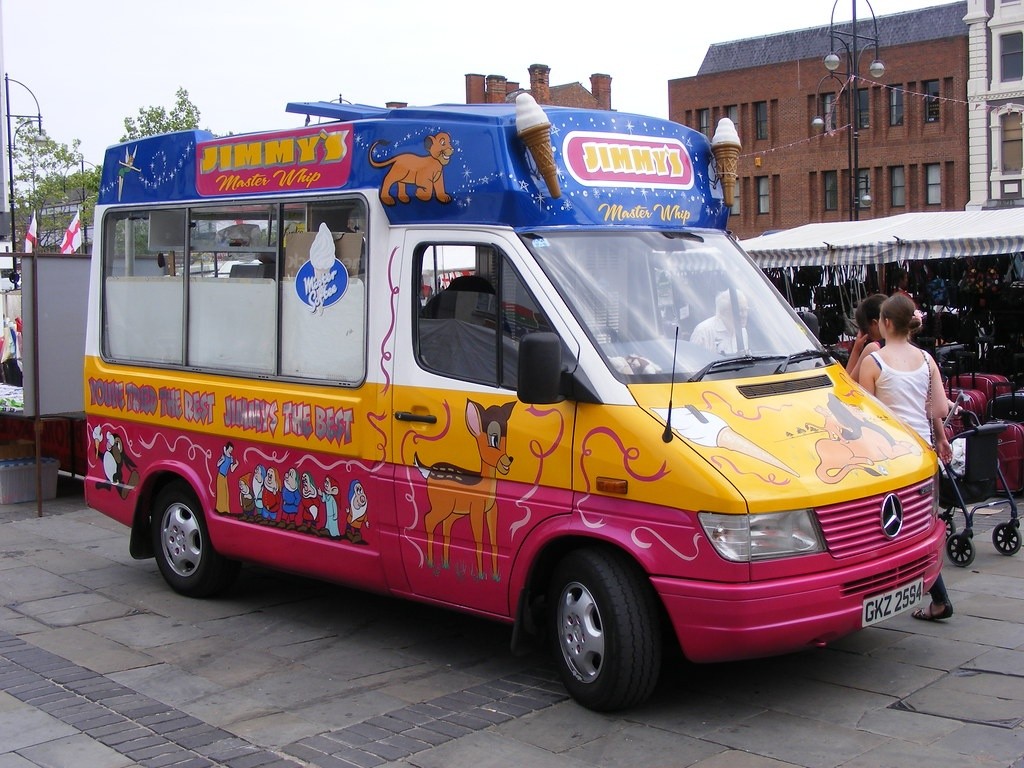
(83, 92), (946, 715)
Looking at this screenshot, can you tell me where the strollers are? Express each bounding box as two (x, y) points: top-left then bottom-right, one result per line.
(934, 389), (1023, 568)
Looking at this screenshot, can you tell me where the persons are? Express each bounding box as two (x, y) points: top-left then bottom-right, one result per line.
(845, 294), (952, 465)
(946, 398), (963, 419)
(858, 294), (954, 620)
(690, 288), (749, 358)
(798, 311), (818, 338)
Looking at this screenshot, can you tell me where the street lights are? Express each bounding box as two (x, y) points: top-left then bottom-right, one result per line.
(3, 72), (48, 291)
(813, 0), (885, 222)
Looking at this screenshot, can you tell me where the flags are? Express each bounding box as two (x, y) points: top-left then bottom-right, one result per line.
(59, 210), (82, 255)
(25, 210), (37, 253)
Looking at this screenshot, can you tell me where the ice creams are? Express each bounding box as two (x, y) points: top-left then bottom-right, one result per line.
(709, 117), (741, 207)
(308, 222), (334, 316)
(515, 93), (560, 197)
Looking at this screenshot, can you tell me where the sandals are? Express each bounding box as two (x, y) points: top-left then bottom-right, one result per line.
(912, 601), (954, 621)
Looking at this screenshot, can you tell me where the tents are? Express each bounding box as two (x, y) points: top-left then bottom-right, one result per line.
(666, 207), (1024, 341)
